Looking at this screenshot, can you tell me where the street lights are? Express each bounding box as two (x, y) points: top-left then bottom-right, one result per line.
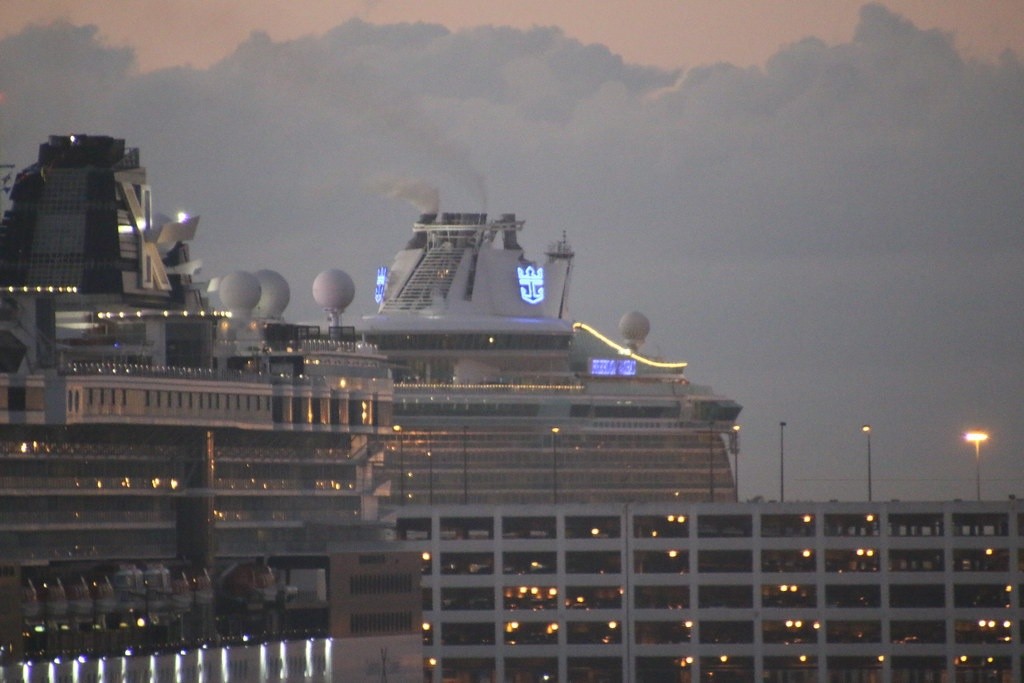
(393, 424), (407, 504)
(779, 422), (787, 503)
(733, 423), (740, 504)
(965, 432), (988, 498)
(862, 426), (872, 499)
(552, 427), (559, 504)
